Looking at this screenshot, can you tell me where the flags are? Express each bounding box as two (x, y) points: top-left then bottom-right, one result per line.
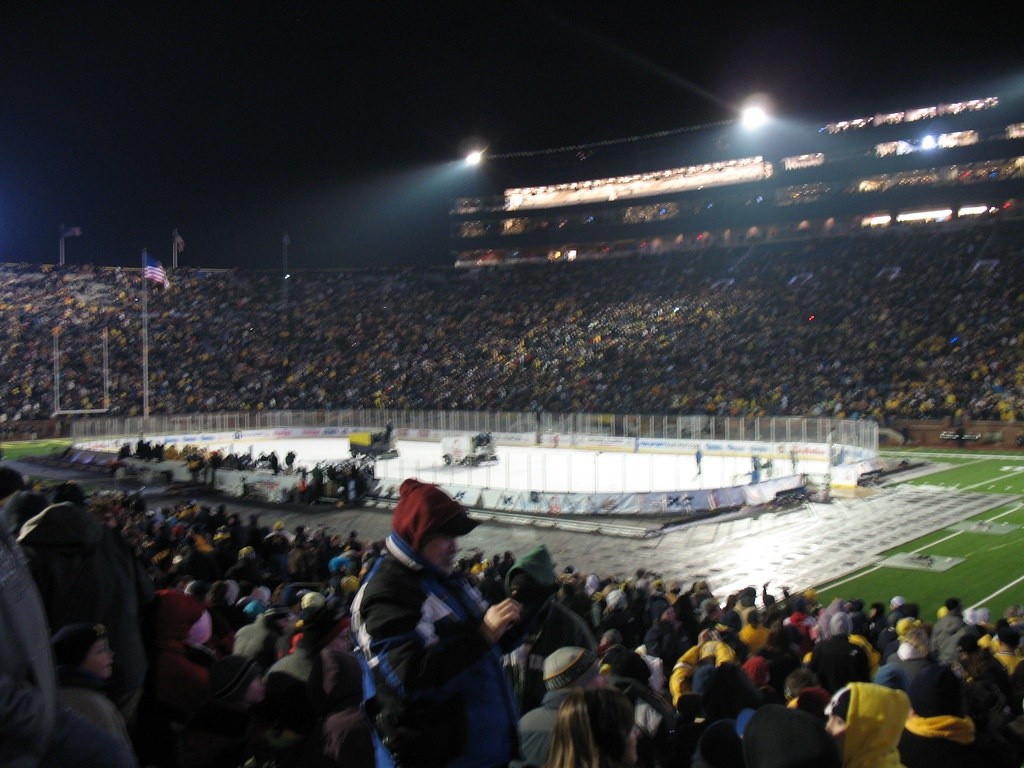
(61, 226), (83, 241)
(143, 257), (170, 288)
(175, 232), (186, 250)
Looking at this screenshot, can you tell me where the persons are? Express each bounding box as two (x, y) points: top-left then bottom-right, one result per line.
(0, 468), (1024, 768)
(749, 455), (761, 482)
(0, 220), (1024, 450)
(696, 449), (703, 474)
(118, 438), (380, 504)
(349, 478), (524, 768)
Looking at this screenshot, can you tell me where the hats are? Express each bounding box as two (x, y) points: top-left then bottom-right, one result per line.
(824, 686), (851, 722)
(437, 512), (480, 537)
(740, 703), (839, 768)
(154, 588), (207, 639)
(890, 595), (905, 608)
(48, 619), (108, 670)
(946, 597), (962, 614)
(329, 555), (352, 577)
(264, 603), (289, 620)
(302, 592), (325, 610)
(650, 596), (672, 619)
(543, 646), (598, 692)
(207, 650), (258, 708)
(608, 590), (629, 610)
(715, 609), (742, 633)
(697, 639), (731, 661)
(998, 627), (1020, 649)
